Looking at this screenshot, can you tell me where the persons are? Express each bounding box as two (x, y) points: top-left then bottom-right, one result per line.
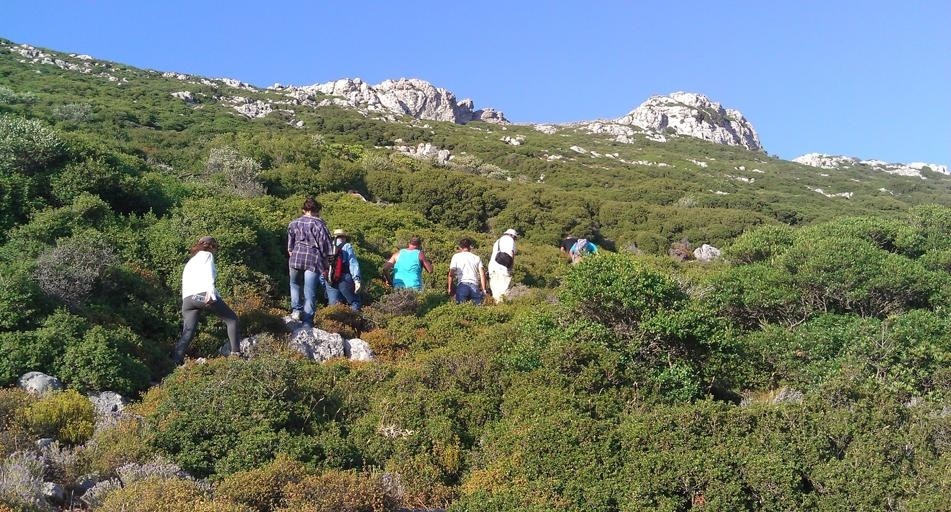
(447, 229), (518, 305)
(561, 234), (598, 262)
(287, 197), (362, 327)
(173, 236), (241, 365)
(383, 237), (432, 291)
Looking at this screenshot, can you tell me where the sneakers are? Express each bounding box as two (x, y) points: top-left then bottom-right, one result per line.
(302, 322), (311, 328)
(288, 309), (302, 320)
(231, 350), (245, 357)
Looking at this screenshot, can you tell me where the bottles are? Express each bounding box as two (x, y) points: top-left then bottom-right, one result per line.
(192, 295), (206, 304)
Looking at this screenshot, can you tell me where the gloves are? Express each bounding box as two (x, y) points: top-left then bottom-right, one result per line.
(353, 280), (361, 293)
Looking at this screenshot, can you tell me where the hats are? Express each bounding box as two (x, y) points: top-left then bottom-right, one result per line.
(331, 229), (348, 238)
(199, 236), (218, 246)
(504, 229), (518, 238)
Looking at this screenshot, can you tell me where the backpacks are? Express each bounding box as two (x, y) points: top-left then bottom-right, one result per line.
(575, 239), (588, 256)
(324, 239), (346, 286)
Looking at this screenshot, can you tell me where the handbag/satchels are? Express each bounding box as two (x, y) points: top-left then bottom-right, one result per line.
(495, 252), (513, 268)
